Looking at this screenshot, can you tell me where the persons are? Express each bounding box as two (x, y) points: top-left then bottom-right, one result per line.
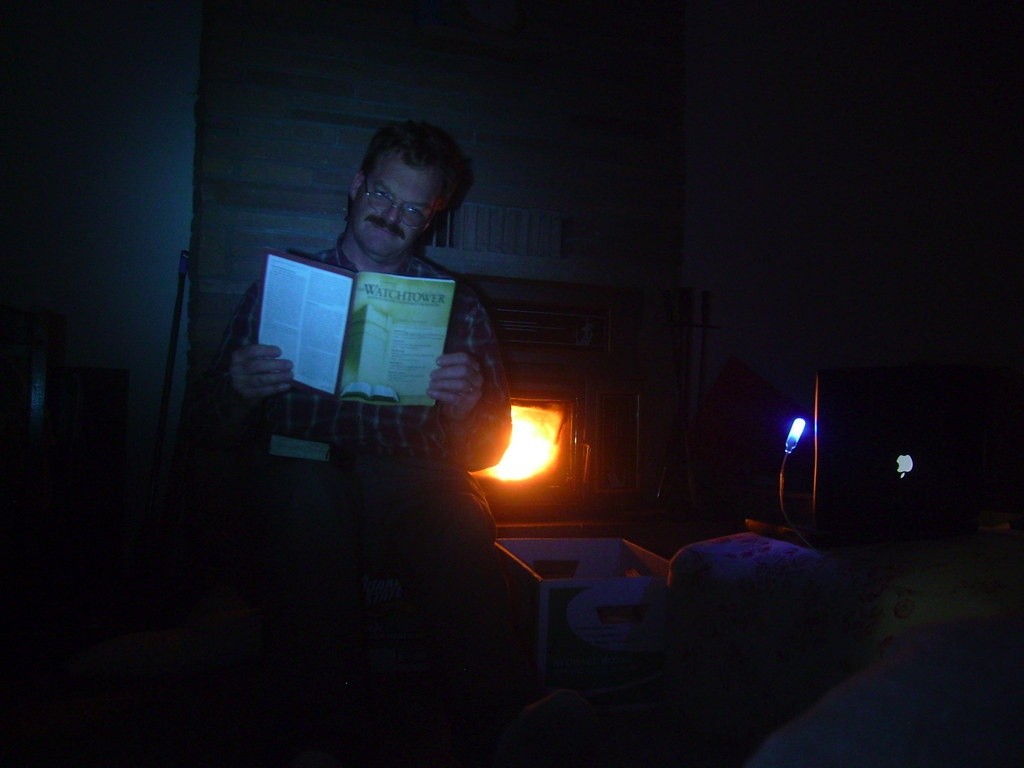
(181, 124), (595, 768)
(746, 610), (1024, 768)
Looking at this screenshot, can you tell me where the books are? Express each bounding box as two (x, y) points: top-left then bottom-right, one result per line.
(257, 248), (458, 403)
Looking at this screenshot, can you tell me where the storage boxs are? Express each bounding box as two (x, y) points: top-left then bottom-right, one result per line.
(490, 534), (676, 708)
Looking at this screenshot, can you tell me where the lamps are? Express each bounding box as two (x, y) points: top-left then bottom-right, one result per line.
(778, 416), (833, 560)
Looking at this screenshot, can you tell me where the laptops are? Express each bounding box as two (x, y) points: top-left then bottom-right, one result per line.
(745, 360), (982, 544)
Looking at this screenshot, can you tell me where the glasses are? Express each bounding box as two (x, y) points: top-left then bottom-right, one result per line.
(362, 171), (437, 233)
